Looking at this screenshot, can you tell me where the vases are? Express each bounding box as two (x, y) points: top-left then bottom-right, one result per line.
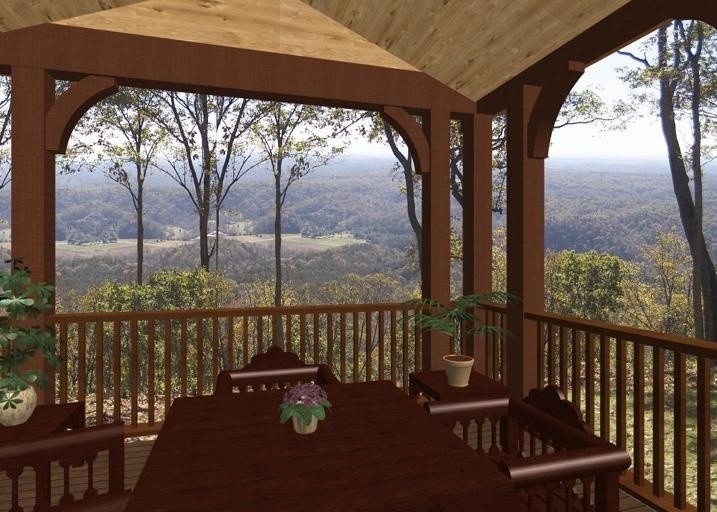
(291, 412), (319, 434)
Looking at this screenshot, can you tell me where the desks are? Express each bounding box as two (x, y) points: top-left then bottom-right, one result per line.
(123, 378), (527, 509)
(2, 402), (84, 451)
(407, 369), (508, 405)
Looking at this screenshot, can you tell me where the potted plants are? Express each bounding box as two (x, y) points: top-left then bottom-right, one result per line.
(395, 288), (522, 387)
(0, 251), (63, 427)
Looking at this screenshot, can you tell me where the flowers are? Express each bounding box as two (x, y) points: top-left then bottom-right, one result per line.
(277, 379), (334, 427)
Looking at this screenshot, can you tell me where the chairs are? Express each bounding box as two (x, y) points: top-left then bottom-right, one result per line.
(422, 383), (631, 512)
(217, 347), (341, 394)
